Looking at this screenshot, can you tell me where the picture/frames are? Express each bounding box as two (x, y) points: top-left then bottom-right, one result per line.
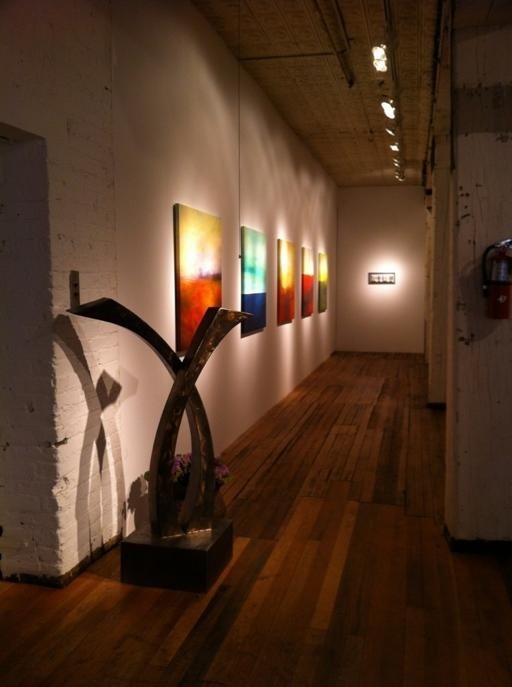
(368, 272), (395, 285)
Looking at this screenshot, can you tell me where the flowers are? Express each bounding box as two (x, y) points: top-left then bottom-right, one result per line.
(170, 453), (229, 487)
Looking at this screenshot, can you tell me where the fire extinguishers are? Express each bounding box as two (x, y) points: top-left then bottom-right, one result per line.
(481, 239), (512, 319)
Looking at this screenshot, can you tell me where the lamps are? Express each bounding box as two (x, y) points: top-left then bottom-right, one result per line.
(372, 43), (408, 183)
